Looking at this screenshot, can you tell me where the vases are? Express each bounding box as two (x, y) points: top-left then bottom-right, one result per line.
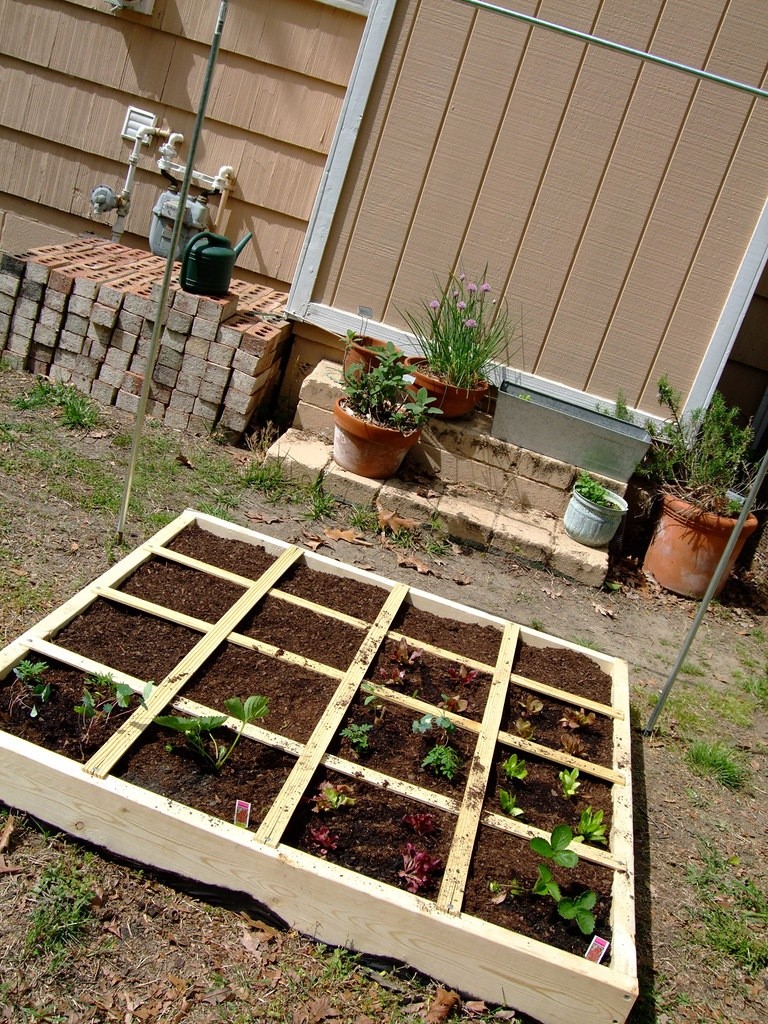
(404, 357), (489, 419)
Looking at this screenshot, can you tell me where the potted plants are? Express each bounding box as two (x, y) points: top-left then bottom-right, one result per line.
(491, 380), (651, 482)
(334, 328), (444, 479)
(341, 328), (400, 388)
(562, 469), (628, 547)
(595, 372), (761, 601)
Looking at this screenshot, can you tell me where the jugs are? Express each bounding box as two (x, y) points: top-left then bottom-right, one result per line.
(178, 232), (252, 296)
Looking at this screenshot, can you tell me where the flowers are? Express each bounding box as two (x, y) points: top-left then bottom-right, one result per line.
(391, 260), (526, 391)
